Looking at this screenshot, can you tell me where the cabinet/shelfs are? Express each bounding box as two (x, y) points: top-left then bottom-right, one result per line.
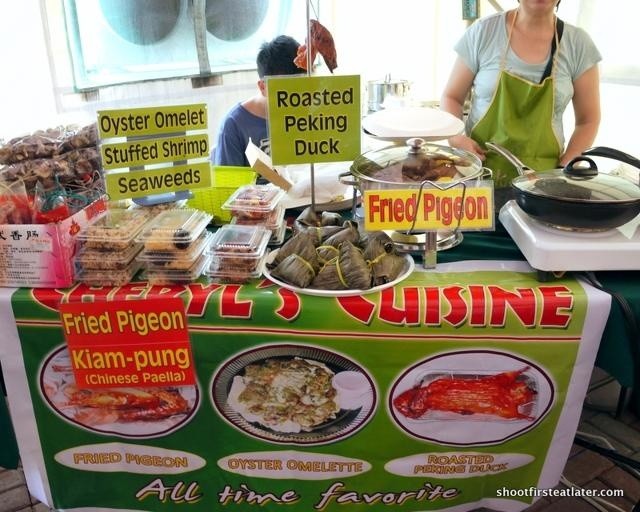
(0, 228), (640, 512)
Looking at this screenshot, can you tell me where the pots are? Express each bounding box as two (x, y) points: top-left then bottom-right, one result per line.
(486, 140), (640, 231)
(336, 134), (484, 192)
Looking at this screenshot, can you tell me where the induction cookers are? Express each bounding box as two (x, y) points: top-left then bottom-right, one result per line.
(498, 198), (640, 281)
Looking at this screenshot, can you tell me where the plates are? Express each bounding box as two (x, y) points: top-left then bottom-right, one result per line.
(37, 338), (558, 447)
(260, 245), (414, 297)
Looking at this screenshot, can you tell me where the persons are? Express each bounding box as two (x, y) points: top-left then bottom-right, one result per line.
(215, 35), (308, 185)
(441, 0), (602, 189)
